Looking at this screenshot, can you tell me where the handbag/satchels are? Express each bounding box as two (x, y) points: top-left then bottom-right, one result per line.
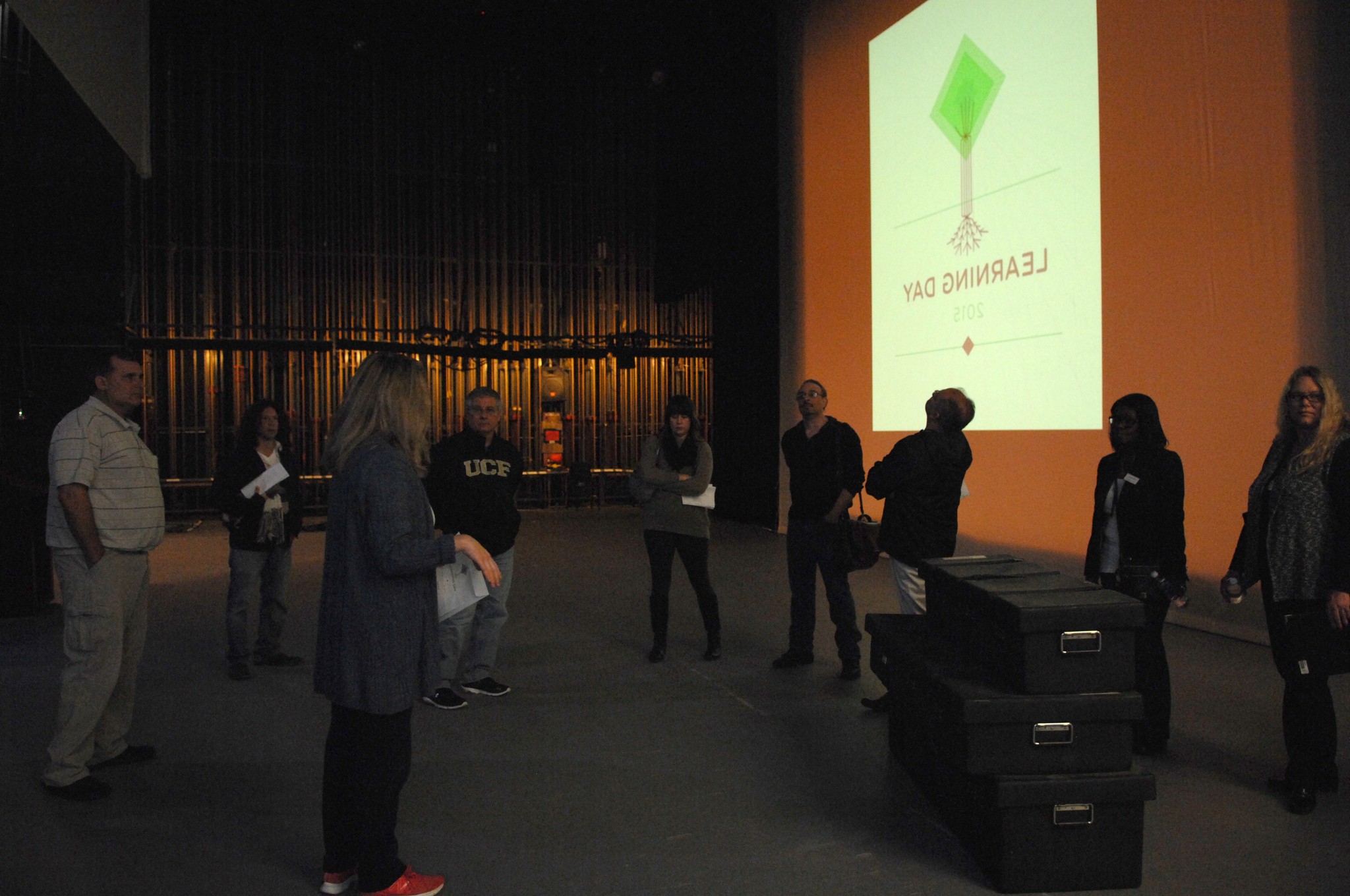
(838, 515), (883, 569)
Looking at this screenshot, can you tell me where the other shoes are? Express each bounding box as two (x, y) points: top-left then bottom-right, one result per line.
(861, 692), (893, 711)
(256, 652), (302, 666)
(229, 662), (247, 680)
(44, 775), (112, 799)
(1290, 785), (1315, 814)
(1268, 770), (1338, 794)
(648, 644), (665, 662)
(97, 745), (154, 767)
(772, 649), (814, 669)
(704, 642), (721, 660)
(841, 660), (860, 679)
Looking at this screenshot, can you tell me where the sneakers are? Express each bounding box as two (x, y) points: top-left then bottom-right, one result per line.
(359, 865), (444, 895)
(320, 864), (358, 894)
(461, 677), (511, 696)
(422, 688), (468, 710)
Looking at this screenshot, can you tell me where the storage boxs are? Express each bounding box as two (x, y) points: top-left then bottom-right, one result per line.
(865, 553), (1156, 894)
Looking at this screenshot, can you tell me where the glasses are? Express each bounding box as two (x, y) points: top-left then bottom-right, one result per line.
(1287, 393), (1322, 402)
(796, 391), (825, 399)
(1109, 414), (1136, 426)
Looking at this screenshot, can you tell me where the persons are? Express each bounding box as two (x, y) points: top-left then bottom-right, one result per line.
(415, 383), (518, 708)
(627, 394), (721, 662)
(773, 380), (865, 679)
(858, 387), (976, 712)
(319, 348), (500, 896)
(212, 396), (302, 678)
(39, 332), (165, 803)
(1078, 392), (1188, 761)
(1220, 365), (1350, 814)
